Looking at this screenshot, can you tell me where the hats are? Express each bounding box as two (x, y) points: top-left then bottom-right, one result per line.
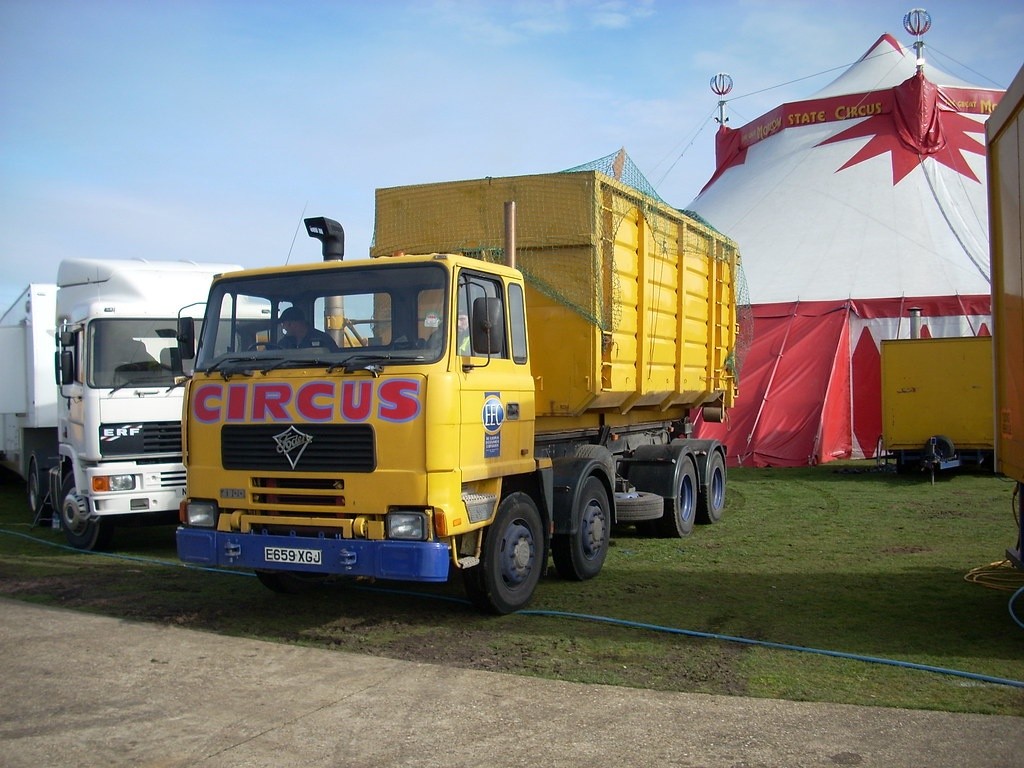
(274, 306), (305, 324)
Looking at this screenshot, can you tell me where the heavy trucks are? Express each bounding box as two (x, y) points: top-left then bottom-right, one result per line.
(159, 169), (742, 615)
(0, 259), (281, 557)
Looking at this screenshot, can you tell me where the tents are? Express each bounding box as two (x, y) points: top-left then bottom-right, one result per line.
(683, 33), (1008, 467)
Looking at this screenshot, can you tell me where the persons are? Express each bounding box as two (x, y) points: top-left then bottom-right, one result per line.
(425, 294), (471, 355)
(273, 308), (343, 352)
(117, 340), (150, 364)
